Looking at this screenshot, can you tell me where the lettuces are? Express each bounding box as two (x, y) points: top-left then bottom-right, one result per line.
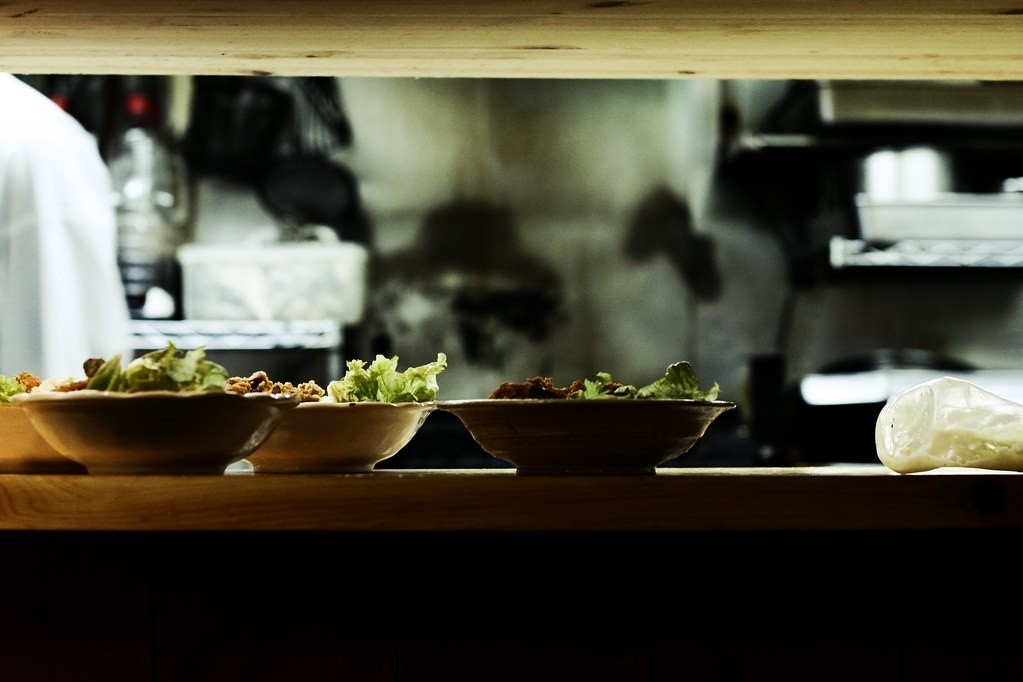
(0, 373), (27, 407)
(83, 340), (232, 390)
(574, 359), (721, 401)
(321, 353), (448, 405)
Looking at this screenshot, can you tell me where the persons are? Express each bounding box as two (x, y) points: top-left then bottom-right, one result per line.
(0, 72), (131, 389)
(182, 82), (379, 379)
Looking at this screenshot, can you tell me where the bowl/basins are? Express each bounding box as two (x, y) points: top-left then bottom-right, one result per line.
(10, 388), (302, 474)
(251, 400), (436, 472)
(438, 401), (736, 475)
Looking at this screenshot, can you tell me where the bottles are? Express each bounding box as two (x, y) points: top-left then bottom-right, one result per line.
(105, 94), (190, 319)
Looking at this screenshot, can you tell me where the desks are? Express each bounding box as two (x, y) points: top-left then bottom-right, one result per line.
(131, 318), (340, 386)
